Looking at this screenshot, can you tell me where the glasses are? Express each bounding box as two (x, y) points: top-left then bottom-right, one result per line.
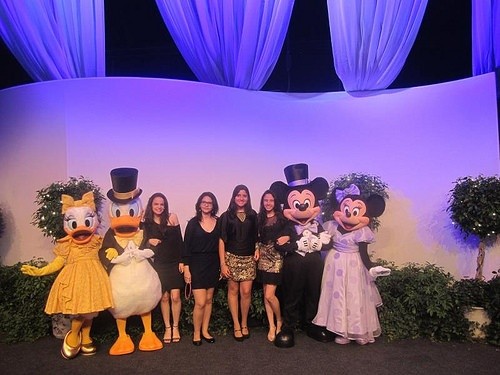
(201, 201), (212, 206)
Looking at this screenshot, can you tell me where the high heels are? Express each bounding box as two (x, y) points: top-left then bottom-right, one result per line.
(163, 327), (172, 344)
(268, 329), (276, 341)
(173, 325), (180, 343)
(276, 321), (282, 335)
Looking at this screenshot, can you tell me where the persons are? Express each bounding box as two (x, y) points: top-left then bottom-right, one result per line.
(183, 192), (220, 345)
(219, 185), (257, 341)
(257, 190), (290, 341)
(146, 194), (183, 343)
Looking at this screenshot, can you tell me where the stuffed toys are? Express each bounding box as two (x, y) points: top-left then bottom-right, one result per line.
(321, 184), (390, 344)
(272, 164), (331, 348)
(98, 169), (163, 355)
(20, 191), (118, 361)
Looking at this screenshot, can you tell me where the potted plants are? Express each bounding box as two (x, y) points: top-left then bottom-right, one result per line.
(51, 313), (71, 339)
(452, 279), (496, 339)
(247, 281), (265, 328)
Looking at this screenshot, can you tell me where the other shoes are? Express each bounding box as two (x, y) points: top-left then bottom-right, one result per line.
(202, 332), (215, 343)
(192, 333), (201, 346)
(242, 326), (249, 339)
(234, 329), (244, 341)
